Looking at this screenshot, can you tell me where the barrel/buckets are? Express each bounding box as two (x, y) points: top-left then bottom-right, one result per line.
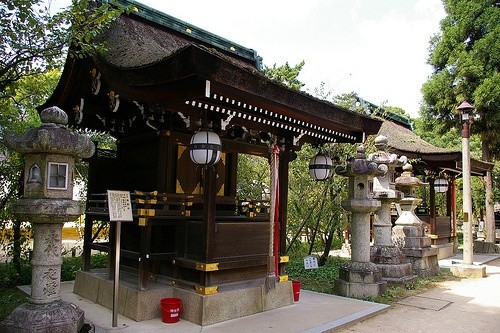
(160, 298), (184, 323)
(292, 280), (300, 301)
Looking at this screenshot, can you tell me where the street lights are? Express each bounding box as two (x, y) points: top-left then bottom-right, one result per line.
(455, 98), (478, 265)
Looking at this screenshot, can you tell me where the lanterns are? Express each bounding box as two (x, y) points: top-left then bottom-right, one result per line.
(188, 131), (223, 167)
(434, 178), (450, 193)
(308, 151), (333, 182)
